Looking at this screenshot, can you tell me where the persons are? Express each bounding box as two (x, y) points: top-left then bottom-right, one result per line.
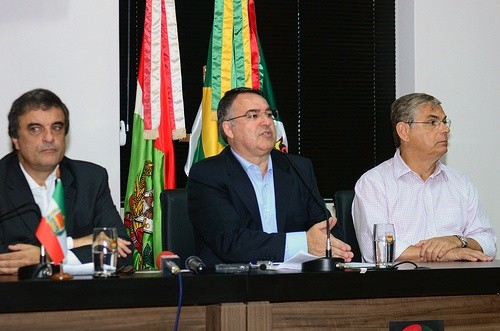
(0, 88), (135, 277)
(184, 86), (354, 266)
(350, 93), (498, 262)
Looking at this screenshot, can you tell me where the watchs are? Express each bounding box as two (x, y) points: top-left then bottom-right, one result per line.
(453, 234), (468, 247)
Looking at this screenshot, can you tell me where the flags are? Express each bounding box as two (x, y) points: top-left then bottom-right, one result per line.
(35, 177), (68, 266)
(173, 0), (288, 179)
(124, 0), (186, 270)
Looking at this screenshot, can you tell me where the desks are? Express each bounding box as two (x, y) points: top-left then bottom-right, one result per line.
(0, 257), (500, 331)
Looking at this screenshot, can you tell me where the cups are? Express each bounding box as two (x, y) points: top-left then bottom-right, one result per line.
(373, 223), (395, 268)
(93, 227), (118, 277)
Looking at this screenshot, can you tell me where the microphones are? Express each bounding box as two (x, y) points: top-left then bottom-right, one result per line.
(279, 144), (345, 272)
(186, 256), (206, 274)
(157, 251), (180, 277)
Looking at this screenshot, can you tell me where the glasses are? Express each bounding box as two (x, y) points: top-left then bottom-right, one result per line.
(229, 109), (278, 121)
(405, 119), (452, 128)
